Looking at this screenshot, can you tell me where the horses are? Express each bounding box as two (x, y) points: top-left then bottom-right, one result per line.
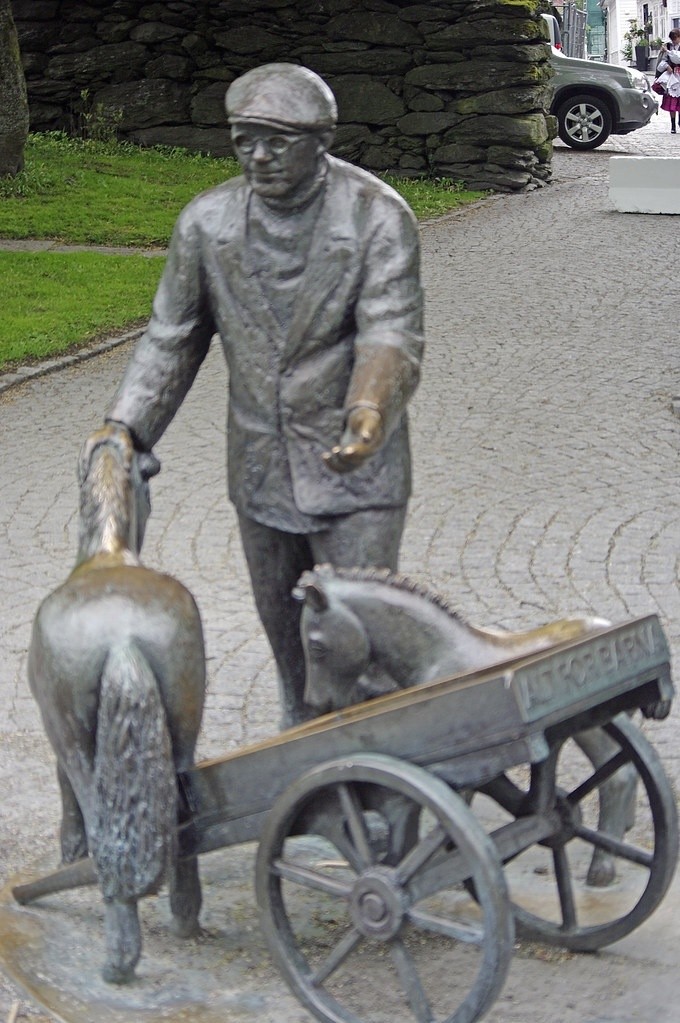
(24, 448), (208, 985)
(294, 561), (639, 887)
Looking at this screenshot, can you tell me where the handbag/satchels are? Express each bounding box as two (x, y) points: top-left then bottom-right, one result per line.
(652, 83), (664, 95)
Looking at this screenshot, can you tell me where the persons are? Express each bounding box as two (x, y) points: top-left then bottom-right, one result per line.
(656, 28), (680, 133)
(77, 63), (428, 733)
(539, 13), (561, 49)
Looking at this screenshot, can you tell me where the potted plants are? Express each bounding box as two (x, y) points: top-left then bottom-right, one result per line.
(634, 22), (662, 71)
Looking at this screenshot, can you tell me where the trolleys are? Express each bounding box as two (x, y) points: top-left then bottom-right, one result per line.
(10, 609), (680, 1023)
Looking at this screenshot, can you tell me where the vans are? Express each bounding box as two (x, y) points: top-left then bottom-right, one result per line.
(540, 14), (562, 52)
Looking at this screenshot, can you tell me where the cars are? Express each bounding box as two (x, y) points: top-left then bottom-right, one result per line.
(551, 46), (659, 149)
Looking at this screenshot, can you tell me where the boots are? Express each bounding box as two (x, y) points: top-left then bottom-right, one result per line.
(671, 117), (676, 133)
(678, 114), (680, 126)
